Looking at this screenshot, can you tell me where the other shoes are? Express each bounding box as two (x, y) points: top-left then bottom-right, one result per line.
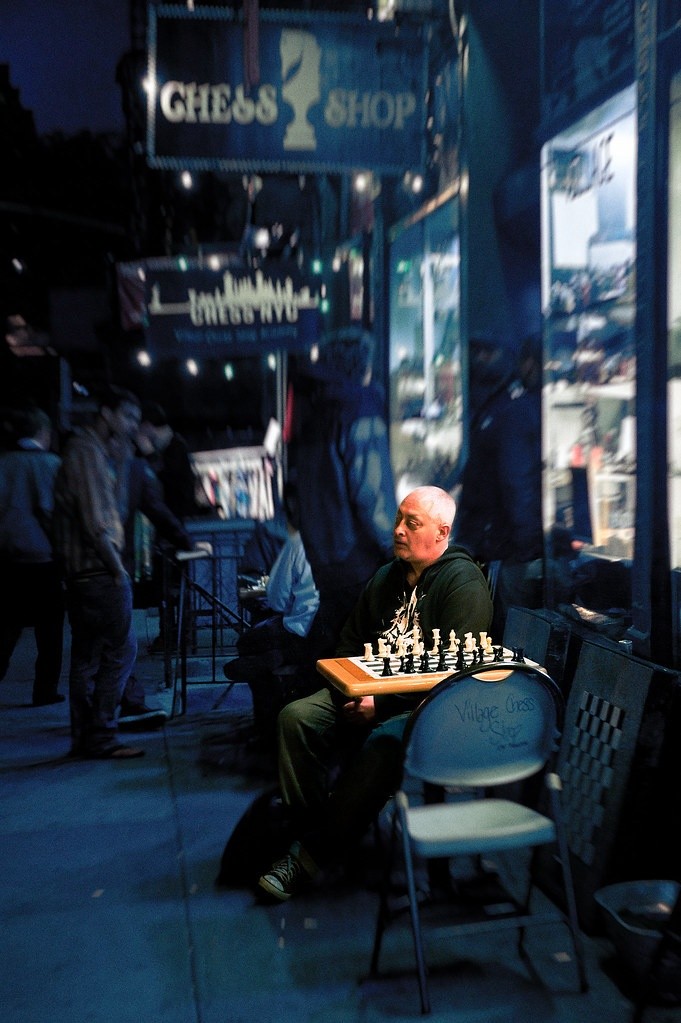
(32, 695), (65, 705)
(224, 642), (296, 682)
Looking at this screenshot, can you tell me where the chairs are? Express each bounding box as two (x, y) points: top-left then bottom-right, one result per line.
(338, 544), (506, 864)
(371, 662), (589, 1017)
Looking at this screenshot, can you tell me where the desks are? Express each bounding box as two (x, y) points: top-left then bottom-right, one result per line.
(316, 644), (552, 913)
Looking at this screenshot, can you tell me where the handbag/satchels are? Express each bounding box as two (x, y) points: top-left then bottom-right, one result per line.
(236, 615), (283, 656)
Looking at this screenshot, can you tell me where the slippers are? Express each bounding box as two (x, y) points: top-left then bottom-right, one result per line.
(82, 744), (145, 760)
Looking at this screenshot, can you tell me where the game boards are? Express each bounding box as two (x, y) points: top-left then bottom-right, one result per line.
(347, 646), (540, 679)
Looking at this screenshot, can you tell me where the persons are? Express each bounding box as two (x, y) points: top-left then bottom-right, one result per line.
(259, 485), (494, 902)
(285, 328), (400, 630)
(0, 384), (213, 761)
(224, 498), (322, 736)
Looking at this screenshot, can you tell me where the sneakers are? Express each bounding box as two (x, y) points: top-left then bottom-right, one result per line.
(119, 701), (168, 730)
(260, 854), (303, 900)
